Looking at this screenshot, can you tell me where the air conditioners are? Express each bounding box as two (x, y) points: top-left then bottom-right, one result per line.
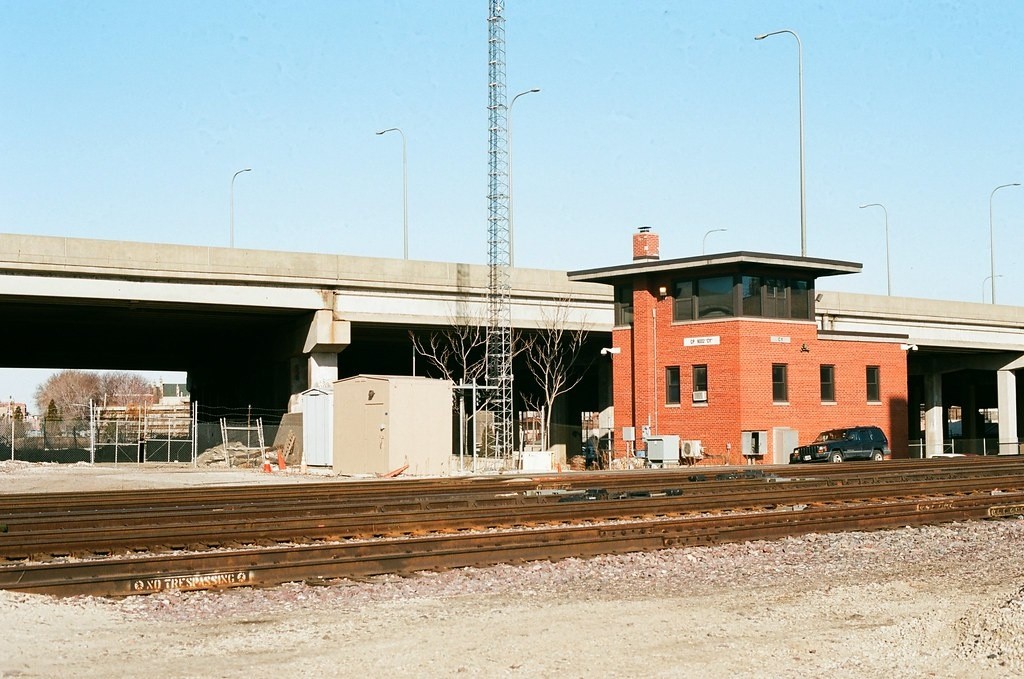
(693, 391), (707, 401)
(681, 440), (703, 458)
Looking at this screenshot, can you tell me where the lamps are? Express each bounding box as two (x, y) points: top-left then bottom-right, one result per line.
(814, 294), (823, 302)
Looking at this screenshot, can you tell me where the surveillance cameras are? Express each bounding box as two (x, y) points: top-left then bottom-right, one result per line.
(601, 348), (607, 356)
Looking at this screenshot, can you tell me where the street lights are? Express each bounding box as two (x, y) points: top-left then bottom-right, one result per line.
(754, 28), (807, 257)
(508, 87), (541, 268)
(374, 127), (409, 261)
(981, 274), (1003, 304)
(230, 168), (252, 249)
(860, 203), (891, 296)
(990, 182), (1024, 304)
(702, 228), (727, 255)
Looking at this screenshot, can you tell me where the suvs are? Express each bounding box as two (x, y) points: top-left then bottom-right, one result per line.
(788, 425), (892, 464)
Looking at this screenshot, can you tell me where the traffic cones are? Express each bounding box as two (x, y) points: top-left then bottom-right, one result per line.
(277, 449), (287, 470)
(374, 464), (409, 479)
(556, 463), (562, 474)
(260, 450), (273, 473)
